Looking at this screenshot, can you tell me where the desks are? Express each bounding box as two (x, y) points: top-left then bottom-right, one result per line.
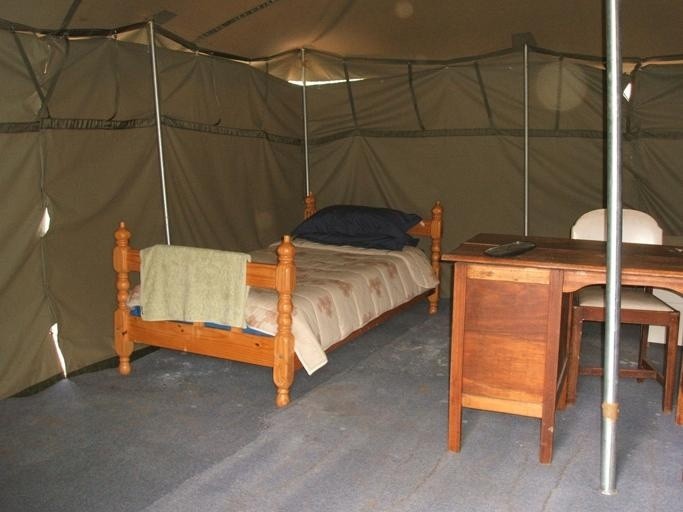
(440, 231), (683, 464)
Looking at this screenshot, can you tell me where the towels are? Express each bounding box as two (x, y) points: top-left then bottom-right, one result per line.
(136, 243), (250, 330)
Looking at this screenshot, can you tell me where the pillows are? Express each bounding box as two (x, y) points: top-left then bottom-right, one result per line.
(291, 203), (421, 250)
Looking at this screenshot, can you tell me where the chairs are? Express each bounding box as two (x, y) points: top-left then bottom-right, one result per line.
(564, 206), (682, 415)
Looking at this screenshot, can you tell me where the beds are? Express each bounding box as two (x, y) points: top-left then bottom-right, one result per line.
(110, 190), (444, 409)
